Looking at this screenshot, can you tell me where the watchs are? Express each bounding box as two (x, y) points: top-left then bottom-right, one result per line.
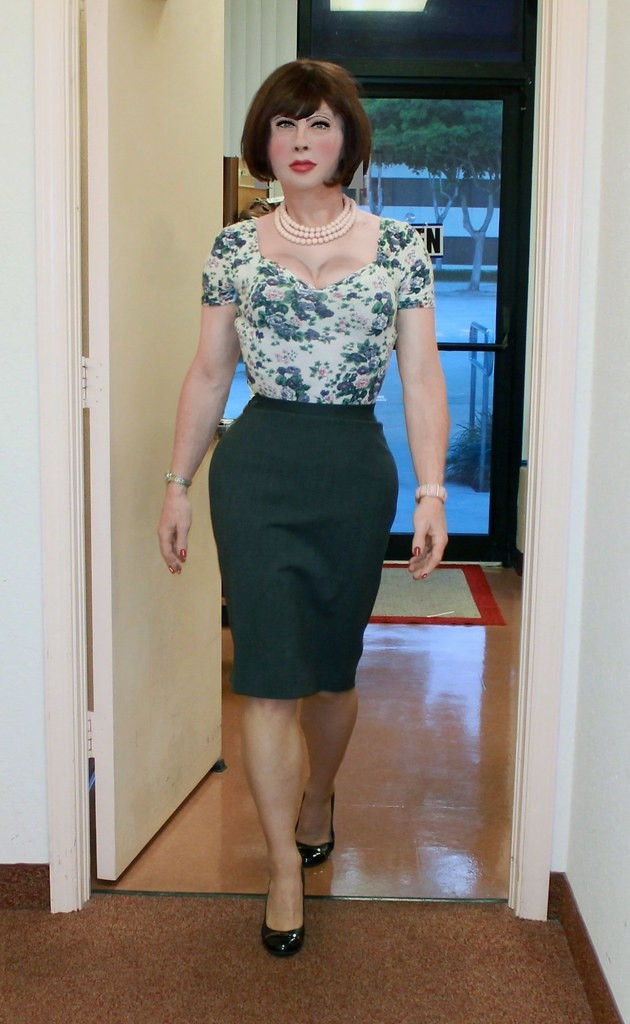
(164, 471), (193, 488)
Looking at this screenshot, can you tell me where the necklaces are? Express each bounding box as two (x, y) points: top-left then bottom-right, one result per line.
(269, 190), (364, 244)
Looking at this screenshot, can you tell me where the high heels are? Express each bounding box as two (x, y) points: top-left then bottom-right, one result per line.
(291, 781), (338, 869)
(261, 859), (308, 959)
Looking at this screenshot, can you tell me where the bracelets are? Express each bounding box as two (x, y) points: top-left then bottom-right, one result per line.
(411, 482), (451, 504)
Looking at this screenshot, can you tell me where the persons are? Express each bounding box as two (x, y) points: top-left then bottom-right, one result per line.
(148, 52), (450, 961)
